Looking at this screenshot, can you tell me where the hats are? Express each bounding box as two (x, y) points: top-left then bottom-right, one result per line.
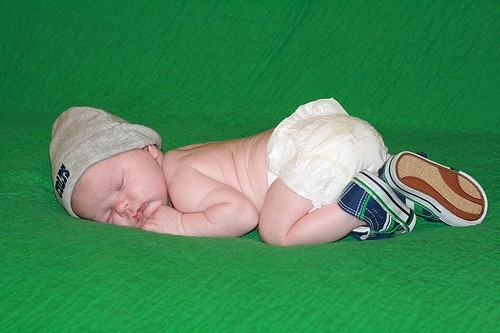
(49, 106), (161, 219)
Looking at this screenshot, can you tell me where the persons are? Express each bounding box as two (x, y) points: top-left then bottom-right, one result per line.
(49, 99), (490, 246)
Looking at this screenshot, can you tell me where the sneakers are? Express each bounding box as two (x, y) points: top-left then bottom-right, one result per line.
(337, 170), (416, 240)
(378, 151), (488, 228)
